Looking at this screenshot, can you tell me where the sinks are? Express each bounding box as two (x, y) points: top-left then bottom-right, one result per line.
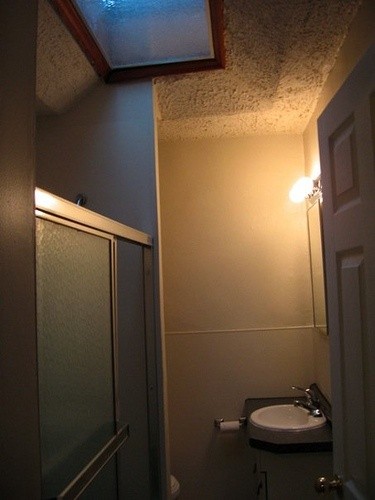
(251, 404), (326, 431)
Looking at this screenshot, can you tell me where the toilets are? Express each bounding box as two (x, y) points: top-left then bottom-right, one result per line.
(171, 474), (180, 499)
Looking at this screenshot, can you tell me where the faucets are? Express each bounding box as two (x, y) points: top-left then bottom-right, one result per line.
(294, 400), (317, 410)
